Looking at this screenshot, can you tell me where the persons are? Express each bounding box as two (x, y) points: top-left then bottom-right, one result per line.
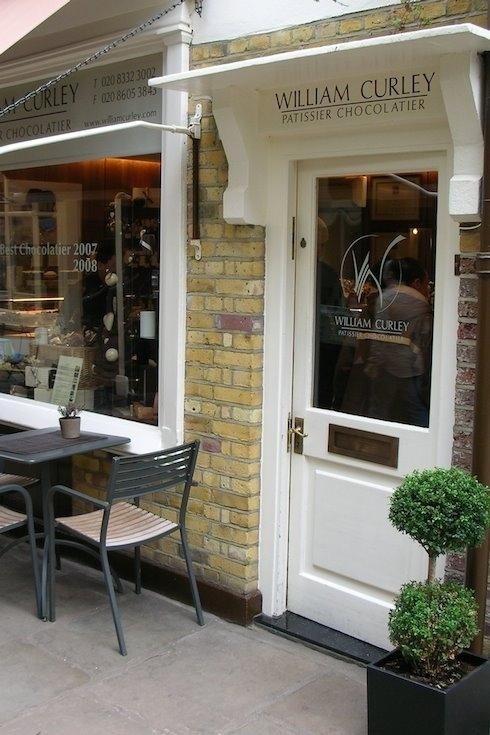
(53, 240), (136, 375)
(334, 255), (434, 389)
(317, 216), (345, 410)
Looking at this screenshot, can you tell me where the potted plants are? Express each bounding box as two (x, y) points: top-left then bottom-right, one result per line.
(367, 464), (489, 735)
(56, 402), (88, 439)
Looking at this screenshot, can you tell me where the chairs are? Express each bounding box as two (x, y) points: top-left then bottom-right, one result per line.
(0, 485), (44, 619)
(0, 471), (62, 569)
(49, 439), (205, 657)
